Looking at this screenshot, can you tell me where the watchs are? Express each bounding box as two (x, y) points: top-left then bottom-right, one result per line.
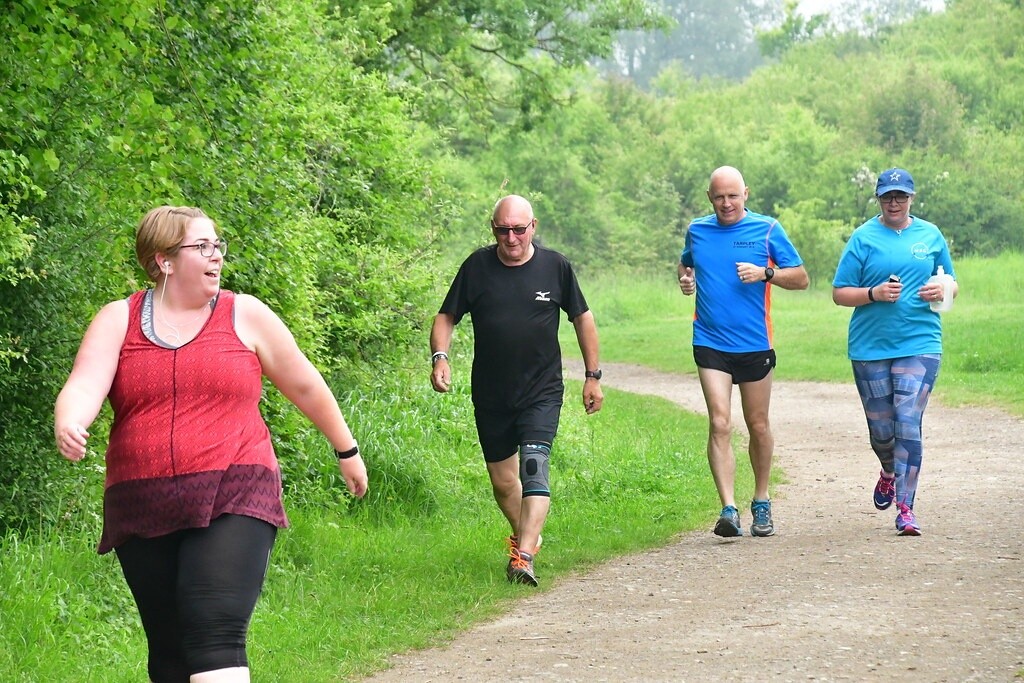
(586, 369), (602, 379)
(762, 266), (774, 283)
(334, 438), (359, 459)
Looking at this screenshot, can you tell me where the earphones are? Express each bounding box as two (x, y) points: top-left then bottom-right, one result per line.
(163, 262), (170, 266)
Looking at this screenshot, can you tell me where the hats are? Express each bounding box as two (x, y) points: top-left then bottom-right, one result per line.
(876, 168), (914, 198)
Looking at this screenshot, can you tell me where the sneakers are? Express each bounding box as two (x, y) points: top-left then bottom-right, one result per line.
(504, 532), (542, 555)
(893, 493), (921, 536)
(714, 505), (742, 537)
(750, 497), (775, 537)
(507, 547), (538, 587)
(874, 469), (895, 510)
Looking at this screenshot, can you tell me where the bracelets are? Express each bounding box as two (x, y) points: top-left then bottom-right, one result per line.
(868, 287), (876, 302)
(432, 351), (449, 368)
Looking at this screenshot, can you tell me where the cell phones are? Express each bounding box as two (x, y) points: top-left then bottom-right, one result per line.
(888, 275), (901, 303)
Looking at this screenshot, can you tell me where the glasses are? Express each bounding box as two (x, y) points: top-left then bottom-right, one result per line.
(493, 219), (534, 235)
(876, 193), (910, 203)
(167, 242), (227, 258)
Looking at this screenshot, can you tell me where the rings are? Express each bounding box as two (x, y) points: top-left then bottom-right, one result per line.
(934, 295), (936, 299)
(890, 294), (893, 298)
(742, 276), (745, 280)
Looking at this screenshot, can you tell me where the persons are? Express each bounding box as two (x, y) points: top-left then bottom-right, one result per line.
(430, 194), (603, 586)
(54, 206), (368, 683)
(832, 167), (959, 535)
(677, 166), (809, 536)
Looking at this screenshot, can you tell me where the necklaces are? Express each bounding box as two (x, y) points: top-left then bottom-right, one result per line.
(893, 228), (902, 236)
